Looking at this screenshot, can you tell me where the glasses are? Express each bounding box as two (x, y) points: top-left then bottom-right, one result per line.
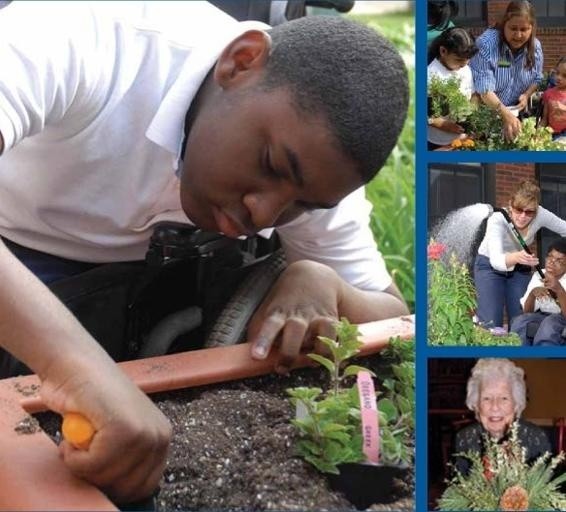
(511, 202), (536, 217)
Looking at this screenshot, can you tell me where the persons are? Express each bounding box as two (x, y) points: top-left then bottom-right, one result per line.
(510, 240), (566, 346)
(427, 0), (459, 67)
(455, 358), (551, 478)
(536, 56), (566, 139)
(474, 178), (566, 330)
(0, 0), (410, 506)
(469, 0), (544, 144)
(427, 27), (481, 150)
